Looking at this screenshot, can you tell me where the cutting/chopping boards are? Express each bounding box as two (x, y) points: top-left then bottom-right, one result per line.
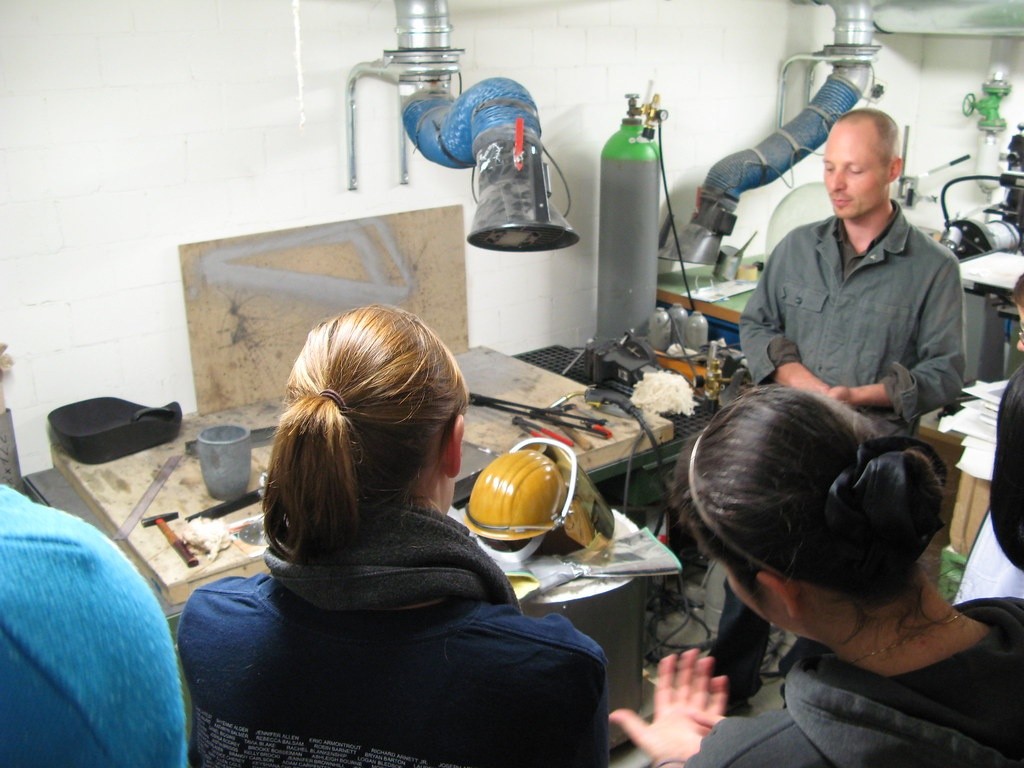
(51, 346), (674, 605)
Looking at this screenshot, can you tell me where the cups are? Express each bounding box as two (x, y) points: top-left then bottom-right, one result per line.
(713, 245), (741, 282)
(197, 424), (251, 500)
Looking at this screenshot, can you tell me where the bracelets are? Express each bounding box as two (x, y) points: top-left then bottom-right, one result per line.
(656, 758), (685, 768)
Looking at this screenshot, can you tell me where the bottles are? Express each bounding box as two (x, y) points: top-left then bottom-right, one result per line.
(648, 303), (708, 351)
(705, 359), (720, 400)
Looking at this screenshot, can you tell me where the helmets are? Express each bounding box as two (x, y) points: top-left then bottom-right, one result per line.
(463, 437), (615, 562)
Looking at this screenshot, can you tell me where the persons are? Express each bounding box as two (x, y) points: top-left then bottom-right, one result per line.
(1013, 274), (1024, 352)
(175, 305), (608, 768)
(0, 482), (189, 768)
(610, 387), (1024, 768)
(990, 363), (1024, 572)
(704, 109), (966, 716)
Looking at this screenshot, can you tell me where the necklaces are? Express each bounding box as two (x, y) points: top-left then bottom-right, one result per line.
(843, 613), (962, 663)
